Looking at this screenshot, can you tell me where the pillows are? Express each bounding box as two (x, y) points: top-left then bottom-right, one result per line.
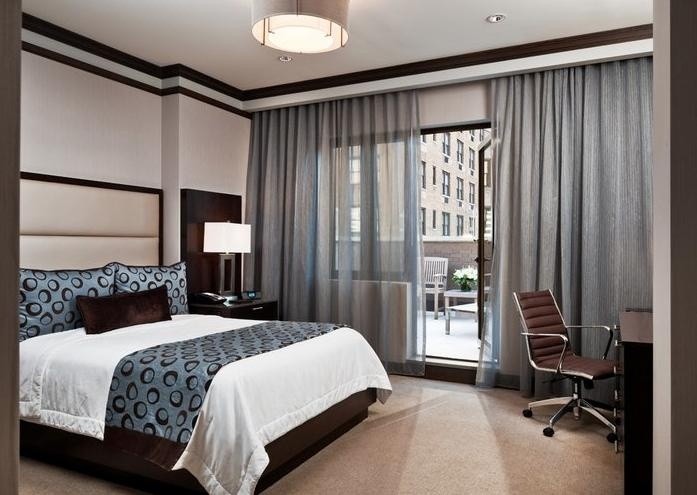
(110, 284), (172, 329)
(115, 260), (188, 314)
(19, 261), (116, 341)
(76, 289), (129, 334)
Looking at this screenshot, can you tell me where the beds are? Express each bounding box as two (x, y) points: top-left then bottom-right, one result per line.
(19, 171), (378, 494)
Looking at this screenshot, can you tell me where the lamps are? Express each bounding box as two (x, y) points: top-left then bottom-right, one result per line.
(202, 221), (251, 301)
(248, 0), (349, 57)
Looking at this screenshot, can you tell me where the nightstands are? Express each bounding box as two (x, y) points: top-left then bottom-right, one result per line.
(194, 298), (278, 320)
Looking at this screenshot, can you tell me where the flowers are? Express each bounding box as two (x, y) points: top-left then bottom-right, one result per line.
(452, 266), (479, 285)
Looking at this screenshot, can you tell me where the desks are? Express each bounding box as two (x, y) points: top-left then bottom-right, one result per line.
(443, 286), (490, 334)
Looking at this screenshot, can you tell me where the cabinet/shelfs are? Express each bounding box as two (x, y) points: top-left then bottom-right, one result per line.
(613, 309), (653, 495)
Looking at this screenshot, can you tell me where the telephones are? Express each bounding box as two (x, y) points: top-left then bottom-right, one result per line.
(196, 291), (226, 304)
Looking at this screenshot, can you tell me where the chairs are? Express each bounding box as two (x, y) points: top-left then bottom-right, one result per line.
(511, 287), (619, 443)
(420, 257), (448, 318)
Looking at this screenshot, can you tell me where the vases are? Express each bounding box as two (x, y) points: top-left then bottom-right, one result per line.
(460, 284), (471, 292)
